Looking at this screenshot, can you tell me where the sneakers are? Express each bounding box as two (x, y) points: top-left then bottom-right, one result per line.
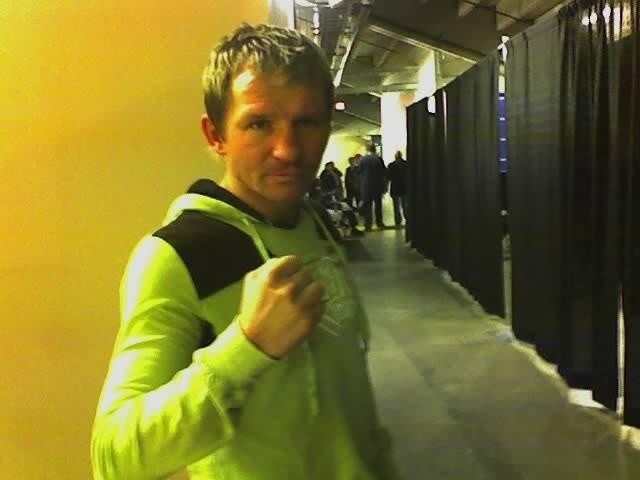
(351, 221), (401, 236)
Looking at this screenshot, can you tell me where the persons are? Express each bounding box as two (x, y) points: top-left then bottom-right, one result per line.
(307, 143), (415, 238)
(88, 22), (388, 479)
